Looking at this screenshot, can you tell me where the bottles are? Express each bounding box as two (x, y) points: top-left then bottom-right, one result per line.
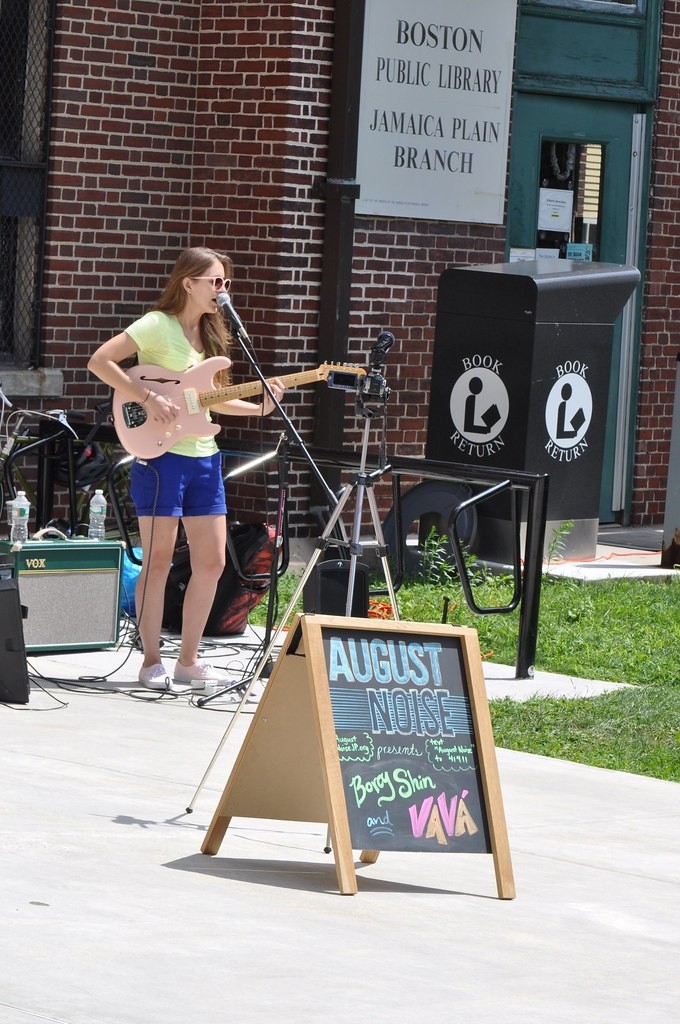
(10, 491), (30, 544)
(88, 489), (107, 542)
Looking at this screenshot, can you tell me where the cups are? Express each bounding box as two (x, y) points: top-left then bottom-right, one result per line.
(5, 500), (31, 540)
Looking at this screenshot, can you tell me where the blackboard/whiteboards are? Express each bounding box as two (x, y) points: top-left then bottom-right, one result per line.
(219, 613), (510, 853)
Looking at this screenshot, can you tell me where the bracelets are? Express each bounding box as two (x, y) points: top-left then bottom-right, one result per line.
(141, 390), (152, 403)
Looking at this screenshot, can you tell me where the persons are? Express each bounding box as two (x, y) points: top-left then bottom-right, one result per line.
(87, 248), (284, 690)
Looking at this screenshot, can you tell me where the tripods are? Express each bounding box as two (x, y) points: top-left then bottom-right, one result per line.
(197, 315), (399, 706)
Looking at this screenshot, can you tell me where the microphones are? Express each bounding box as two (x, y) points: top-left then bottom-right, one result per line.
(217, 293), (252, 344)
(370, 331), (396, 364)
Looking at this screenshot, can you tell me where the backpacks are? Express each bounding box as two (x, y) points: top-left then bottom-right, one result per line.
(160, 521), (281, 637)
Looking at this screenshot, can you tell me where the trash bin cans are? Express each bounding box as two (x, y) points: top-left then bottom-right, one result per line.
(417, 255), (641, 564)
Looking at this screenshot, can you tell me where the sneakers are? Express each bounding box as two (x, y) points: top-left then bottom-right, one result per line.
(174, 659), (231, 686)
(138, 661), (174, 689)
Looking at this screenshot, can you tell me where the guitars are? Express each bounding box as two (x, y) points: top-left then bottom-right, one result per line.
(112, 354), (368, 460)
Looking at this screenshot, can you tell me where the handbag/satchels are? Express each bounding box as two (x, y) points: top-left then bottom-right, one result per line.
(52, 439), (110, 487)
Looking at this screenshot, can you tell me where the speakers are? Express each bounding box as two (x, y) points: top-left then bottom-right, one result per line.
(0, 537), (126, 702)
(303, 558), (370, 617)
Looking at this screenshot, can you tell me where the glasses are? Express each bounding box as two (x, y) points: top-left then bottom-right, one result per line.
(191, 276), (231, 293)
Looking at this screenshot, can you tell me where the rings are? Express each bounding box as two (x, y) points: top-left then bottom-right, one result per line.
(274, 393), (276, 397)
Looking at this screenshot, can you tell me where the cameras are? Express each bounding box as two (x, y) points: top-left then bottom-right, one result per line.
(326, 371), (391, 403)
